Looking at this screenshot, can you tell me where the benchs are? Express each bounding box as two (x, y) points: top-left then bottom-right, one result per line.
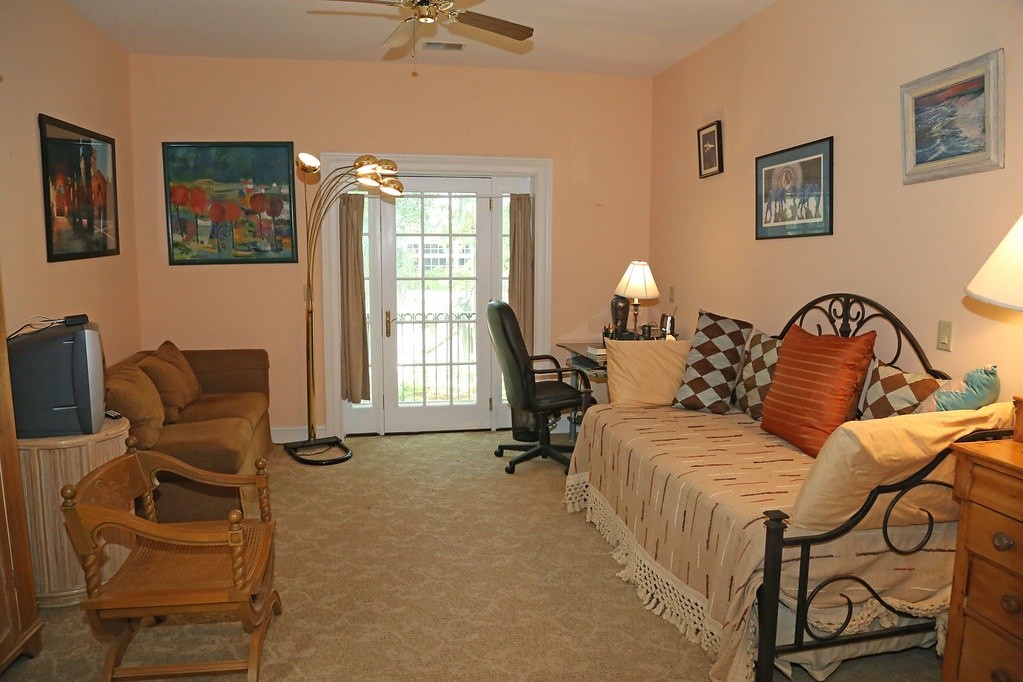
(588, 293), (1014, 682)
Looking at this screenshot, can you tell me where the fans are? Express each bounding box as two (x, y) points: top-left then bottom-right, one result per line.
(329, 0), (535, 49)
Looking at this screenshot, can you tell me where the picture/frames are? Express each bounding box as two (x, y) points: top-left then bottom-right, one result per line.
(38, 112), (119, 262)
(899, 49), (1005, 186)
(756, 137), (834, 241)
(161, 140), (301, 265)
(697, 120), (724, 179)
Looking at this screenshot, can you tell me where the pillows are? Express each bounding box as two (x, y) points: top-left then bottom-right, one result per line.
(671, 307), (755, 415)
(105, 366), (165, 450)
(912, 365), (1001, 414)
(135, 339), (202, 425)
(729, 329), (783, 422)
(760, 324), (879, 458)
(860, 357), (955, 422)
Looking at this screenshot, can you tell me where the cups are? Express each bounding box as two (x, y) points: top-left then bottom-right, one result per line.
(602, 332), (613, 346)
(642, 325), (651, 339)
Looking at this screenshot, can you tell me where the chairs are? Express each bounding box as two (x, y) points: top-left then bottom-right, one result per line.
(486, 301), (598, 476)
(58, 436), (282, 682)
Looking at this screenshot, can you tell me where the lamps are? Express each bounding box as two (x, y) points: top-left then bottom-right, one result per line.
(283, 152), (405, 465)
(615, 258), (661, 329)
(386, 0), (465, 59)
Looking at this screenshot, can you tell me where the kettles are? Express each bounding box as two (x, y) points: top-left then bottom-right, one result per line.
(660, 313), (674, 335)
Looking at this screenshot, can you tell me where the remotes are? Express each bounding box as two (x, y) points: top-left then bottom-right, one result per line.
(104, 410), (122, 419)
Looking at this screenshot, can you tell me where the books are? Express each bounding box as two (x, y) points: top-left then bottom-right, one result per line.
(587, 342), (607, 356)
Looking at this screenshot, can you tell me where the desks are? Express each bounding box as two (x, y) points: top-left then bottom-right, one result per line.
(17, 417), (136, 622)
(556, 342), (609, 444)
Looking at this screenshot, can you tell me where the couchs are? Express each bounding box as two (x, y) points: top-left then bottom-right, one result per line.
(103, 348), (275, 524)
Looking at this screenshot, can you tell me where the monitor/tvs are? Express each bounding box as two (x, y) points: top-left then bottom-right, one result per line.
(8, 321), (104, 439)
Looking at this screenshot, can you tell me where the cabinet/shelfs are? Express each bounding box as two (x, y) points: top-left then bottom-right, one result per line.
(943, 439), (1023, 682)
(0, 282), (45, 673)
